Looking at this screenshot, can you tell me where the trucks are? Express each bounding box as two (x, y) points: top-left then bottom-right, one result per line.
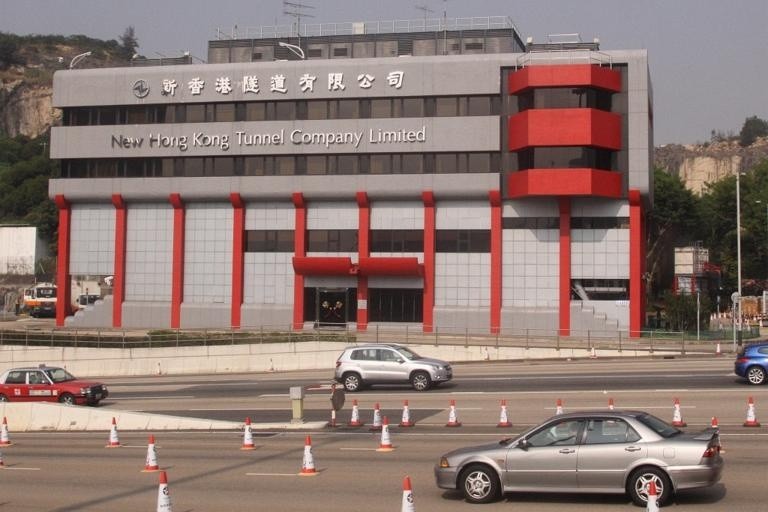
(22, 281), (57, 318)
(76, 292), (100, 310)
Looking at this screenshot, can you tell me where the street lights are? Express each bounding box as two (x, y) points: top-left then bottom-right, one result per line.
(732, 170), (745, 348)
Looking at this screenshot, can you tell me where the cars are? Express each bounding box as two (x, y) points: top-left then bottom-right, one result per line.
(430, 409), (725, 509)
(0, 363), (111, 408)
(731, 342), (767, 386)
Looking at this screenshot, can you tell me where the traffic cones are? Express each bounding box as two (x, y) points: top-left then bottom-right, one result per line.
(606, 397), (616, 410)
(400, 476), (416, 512)
(347, 398), (363, 427)
(239, 416), (255, 451)
(713, 343), (721, 357)
(494, 398), (512, 429)
(0, 451), (8, 470)
(154, 470), (175, 512)
(708, 308), (763, 333)
(374, 415), (396, 453)
(0, 416), (15, 448)
(443, 399), (465, 428)
(137, 432), (161, 474)
(103, 415), (122, 449)
(639, 477), (650, 512)
(397, 399), (415, 428)
(668, 399), (687, 429)
(553, 398), (563, 416)
(739, 397), (761, 427)
(708, 412), (725, 455)
(296, 434), (322, 477)
(368, 402), (386, 432)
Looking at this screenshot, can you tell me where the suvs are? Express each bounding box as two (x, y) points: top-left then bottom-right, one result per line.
(332, 340), (455, 392)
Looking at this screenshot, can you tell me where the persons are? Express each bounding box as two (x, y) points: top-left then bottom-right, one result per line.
(15, 296), (21, 316)
(547, 423), (580, 446)
